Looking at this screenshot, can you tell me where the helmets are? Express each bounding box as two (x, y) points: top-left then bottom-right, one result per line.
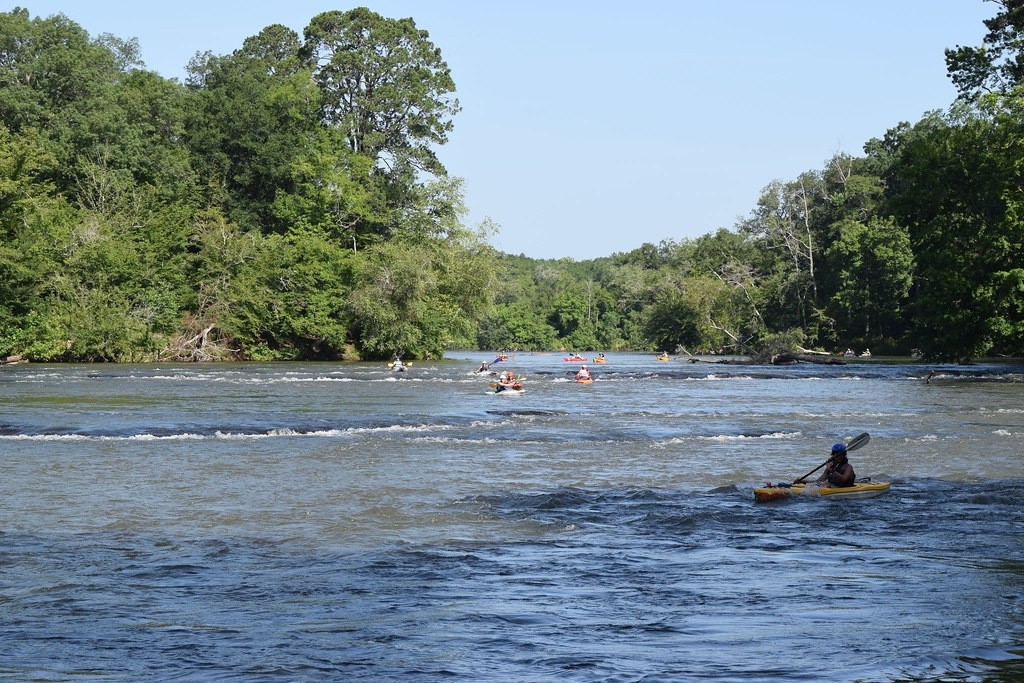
(831, 442), (846, 452)
(500, 375), (506, 379)
(508, 372), (512, 377)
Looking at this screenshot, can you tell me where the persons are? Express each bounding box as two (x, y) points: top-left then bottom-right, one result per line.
(662, 351), (669, 358)
(793, 443), (856, 488)
(476, 360), (490, 373)
(570, 351), (581, 358)
(394, 357), (402, 366)
(576, 365), (592, 379)
(496, 372), (517, 392)
(598, 353), (605, 358)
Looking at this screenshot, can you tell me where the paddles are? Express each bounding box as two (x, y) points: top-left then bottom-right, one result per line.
(489, 377), (527, 387)
(570, 353), (575, 356)
(388, 361), (413, 367)
(488, 358), (502, 367)
(793, 432), (872, 484)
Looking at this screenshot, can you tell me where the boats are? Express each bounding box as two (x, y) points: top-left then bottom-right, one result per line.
(844, 353), (855, 359)
(563, 356), (588, 361)
(496, 382), (522, 392)
(657, 354), (670, 361)
(576, 377), (593, 385)
(861, 353), (872, 359)
(391, 364), (407, 373)
(477, 370), (497, 377)
(593, 357), (608, 363)
(753, 475), (891, 504)
